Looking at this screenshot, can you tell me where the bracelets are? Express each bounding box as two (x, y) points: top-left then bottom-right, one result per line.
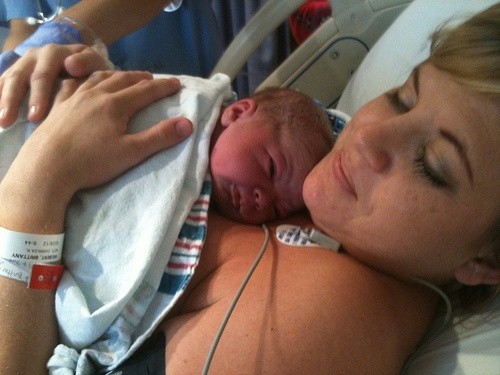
(0, 227), (64, 290)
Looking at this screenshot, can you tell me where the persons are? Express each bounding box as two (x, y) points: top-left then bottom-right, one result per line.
(0, 0), (335, 225)
(0, 0), (500, 375)
